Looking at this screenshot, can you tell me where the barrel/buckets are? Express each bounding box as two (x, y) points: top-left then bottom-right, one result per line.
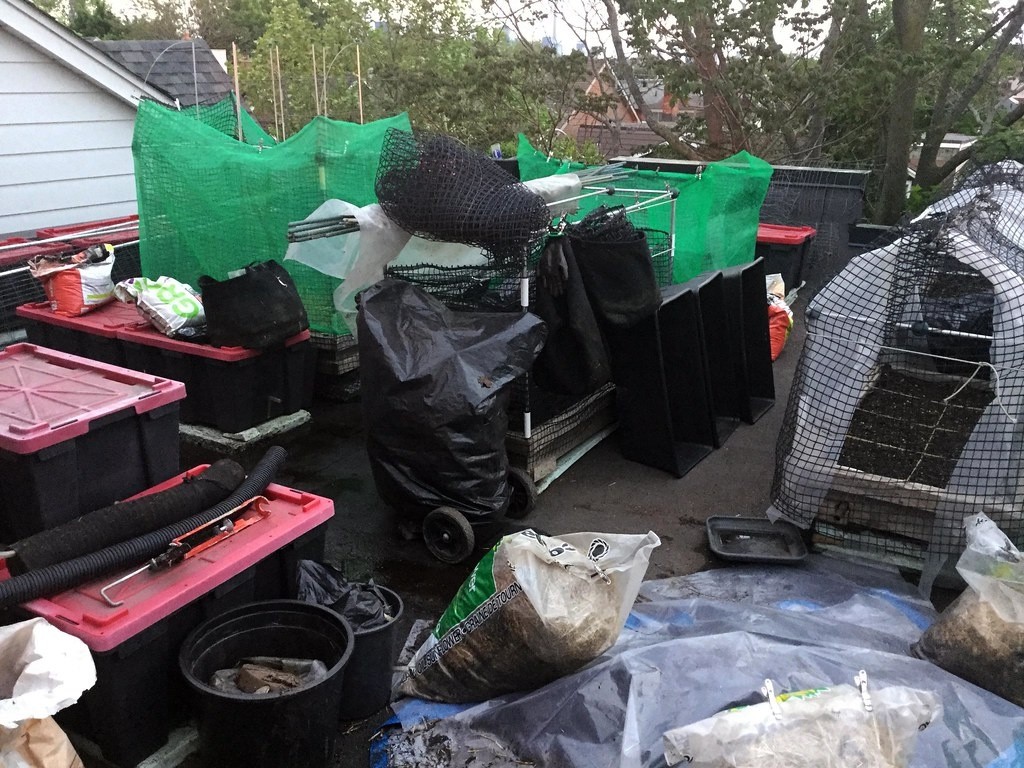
(178, 598), (354, 767)
(339, 582), (404, 721)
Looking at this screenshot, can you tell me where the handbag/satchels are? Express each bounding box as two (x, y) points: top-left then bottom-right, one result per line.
(30, 242), (116, 316)
(114, 274), (208, 338)
(200, 259), (309, 350)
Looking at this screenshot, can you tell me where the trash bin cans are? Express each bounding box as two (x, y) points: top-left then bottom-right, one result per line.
(317, 580), (403, 724)
(178, 597), (353, 768)
